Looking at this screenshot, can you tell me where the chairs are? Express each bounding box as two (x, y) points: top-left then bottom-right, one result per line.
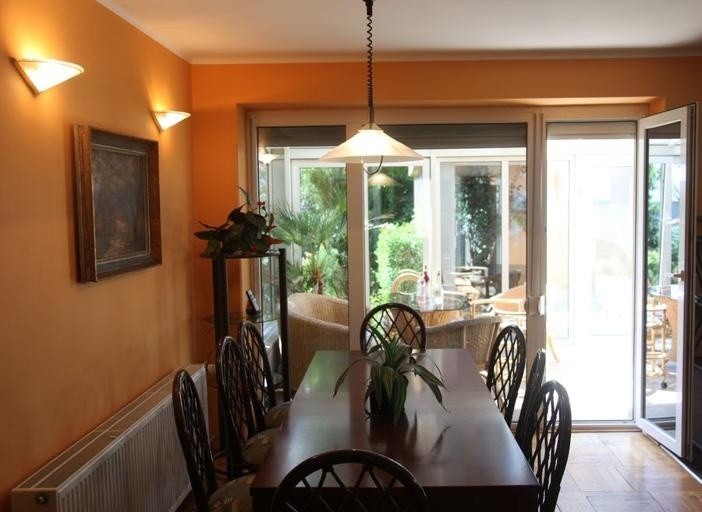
(233, 321), (275, 416)
(275, 290), (502, 387)
(520, 382), (572, 512)
(213, 336), (258, 459)
(273, 450), (428, 511)
(515, 349), (546, 460)
(486, 326), (526, 428)
(169, 370), (219, 510)
(656, 295), (678, 365)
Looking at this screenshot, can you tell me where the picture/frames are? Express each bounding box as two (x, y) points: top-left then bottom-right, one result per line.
(72, 126), (161, 283)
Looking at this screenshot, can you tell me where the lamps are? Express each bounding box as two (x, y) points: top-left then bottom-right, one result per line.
(326, 2), (424, 178)
(151, 106), (192, 132)
(11, 58), (84, 96)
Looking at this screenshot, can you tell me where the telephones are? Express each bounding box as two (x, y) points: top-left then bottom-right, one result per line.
(245, 289), (260, 315)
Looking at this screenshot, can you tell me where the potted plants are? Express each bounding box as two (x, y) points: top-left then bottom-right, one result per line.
(333, 326), (449, 427)
(194, 186), (282, 254)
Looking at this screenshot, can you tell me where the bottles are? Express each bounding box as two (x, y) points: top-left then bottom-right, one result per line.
(417, 264), (444, 305)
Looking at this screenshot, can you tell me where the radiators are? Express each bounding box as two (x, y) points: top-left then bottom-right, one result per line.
(9, 363), (212, 512)
